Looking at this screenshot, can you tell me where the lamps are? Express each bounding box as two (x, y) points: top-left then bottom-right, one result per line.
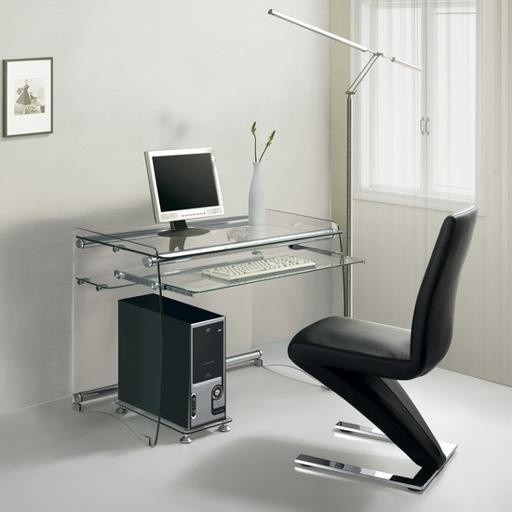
(268, 10), (425, 320)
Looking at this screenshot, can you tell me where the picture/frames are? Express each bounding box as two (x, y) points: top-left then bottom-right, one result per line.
(3, 57), (54, 137)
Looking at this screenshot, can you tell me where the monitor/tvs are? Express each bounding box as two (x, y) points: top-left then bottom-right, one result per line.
(144, 146), (225, 237)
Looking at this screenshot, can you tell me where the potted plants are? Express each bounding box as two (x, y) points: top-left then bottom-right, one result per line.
(248, 120), (276, 226)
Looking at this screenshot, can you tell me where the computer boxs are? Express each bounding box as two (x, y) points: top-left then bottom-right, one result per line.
(118, 292), (226, 429)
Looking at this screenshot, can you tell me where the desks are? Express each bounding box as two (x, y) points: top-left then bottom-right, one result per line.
(72, 208), (365, 448)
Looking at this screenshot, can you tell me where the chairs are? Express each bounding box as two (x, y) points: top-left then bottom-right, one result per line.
(287, 205), (480, 495)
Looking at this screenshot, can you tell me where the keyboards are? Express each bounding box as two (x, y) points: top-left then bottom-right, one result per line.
(202, 255), (316, 284)
